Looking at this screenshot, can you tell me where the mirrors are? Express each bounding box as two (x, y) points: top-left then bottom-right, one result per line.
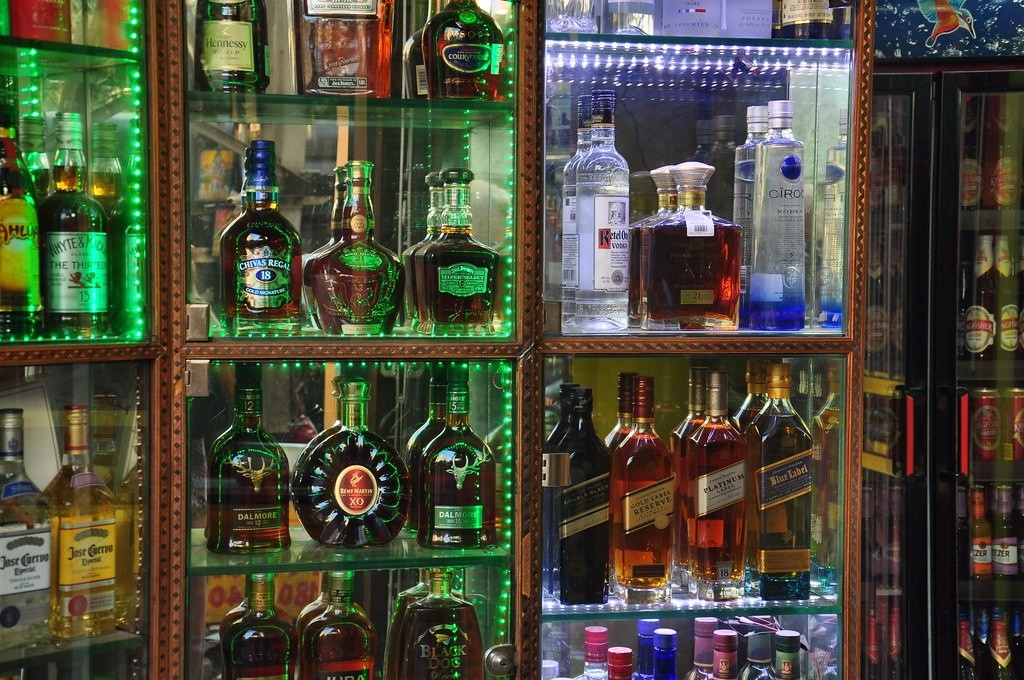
(184, 0), (518, 680)
(0, 0), (149, 680)
(544, 0), (857, 680)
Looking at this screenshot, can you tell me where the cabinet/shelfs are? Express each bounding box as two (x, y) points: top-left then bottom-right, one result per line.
(0, 0), (873, 680)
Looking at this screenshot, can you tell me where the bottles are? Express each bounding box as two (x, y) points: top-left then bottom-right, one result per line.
(291, 382), (412, 546)
(558, 84), (807, 335)
(734, 363), (813, 583)
(221, 139), (303, 336)
(195, 0), (270, 95)
(672, 363), (748, 600)
(407, 360), (498, 548)
(542, 617), (802, 680)
(312, 160), (404, 337)
(963, 92), (1024, 208)
(544, 381), (609, 607)
(220, 569), (485, 680)
(204, 362), (291, 557)
(0, 405), (119, 599)
(611, 374), (674, 588)
(970, 483), (1024, 579)
(0, 105), (111, 338)
(415, 170), (499, 335)
(403, 0), (504, 100)
(294, 0), (394, 98)
(957, 605), (1024, 680)
(961, 234), (1024, 379)
(545, 1), (856, 39)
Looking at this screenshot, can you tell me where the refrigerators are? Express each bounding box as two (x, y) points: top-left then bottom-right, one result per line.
(863, 0), (1024, 680)
